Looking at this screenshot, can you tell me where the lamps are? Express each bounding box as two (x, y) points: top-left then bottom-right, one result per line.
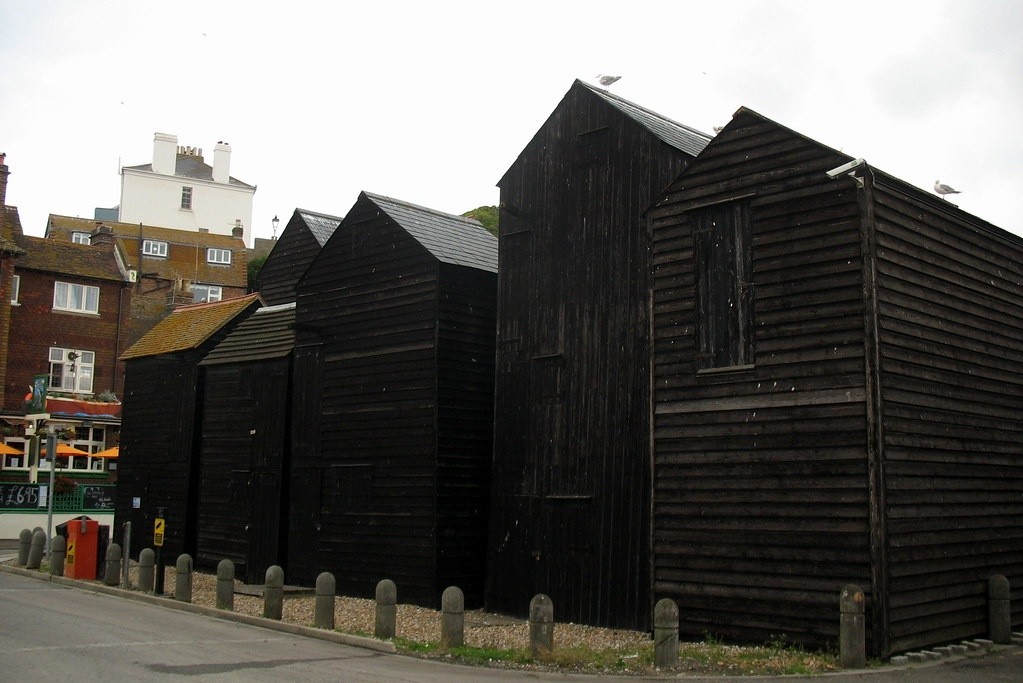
(828, 158), (866, 188)
(272, 215), (279, 241)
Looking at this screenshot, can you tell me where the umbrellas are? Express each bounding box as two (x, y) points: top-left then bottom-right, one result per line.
(0, 441), (27, 455)
(41, 443), (90, 458)
(86, 447), (119, 459)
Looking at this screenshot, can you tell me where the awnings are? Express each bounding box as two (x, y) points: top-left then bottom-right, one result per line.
(46, 395), (121, 418)
